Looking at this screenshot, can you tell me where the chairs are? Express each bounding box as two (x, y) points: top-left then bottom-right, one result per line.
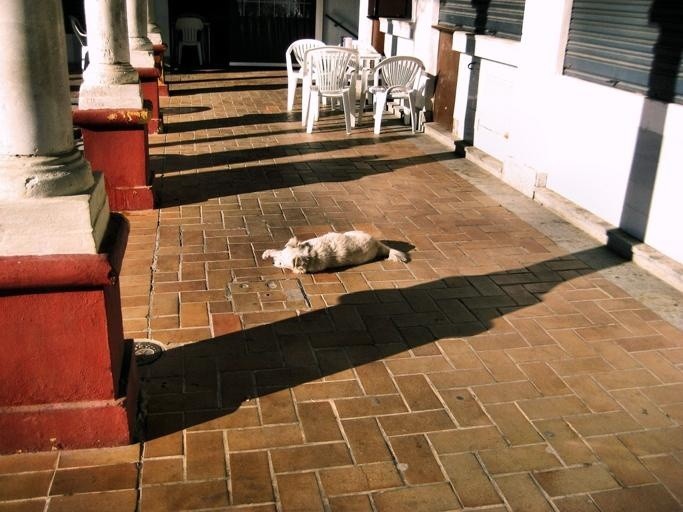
(283, 38), (431, 136)
(174, 17), (205, 65)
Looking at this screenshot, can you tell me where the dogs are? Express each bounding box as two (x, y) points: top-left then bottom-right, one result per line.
(260, 230), (409, 274)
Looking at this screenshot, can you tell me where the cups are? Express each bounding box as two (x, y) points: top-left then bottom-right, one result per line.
(343, 37), (353, 49)
